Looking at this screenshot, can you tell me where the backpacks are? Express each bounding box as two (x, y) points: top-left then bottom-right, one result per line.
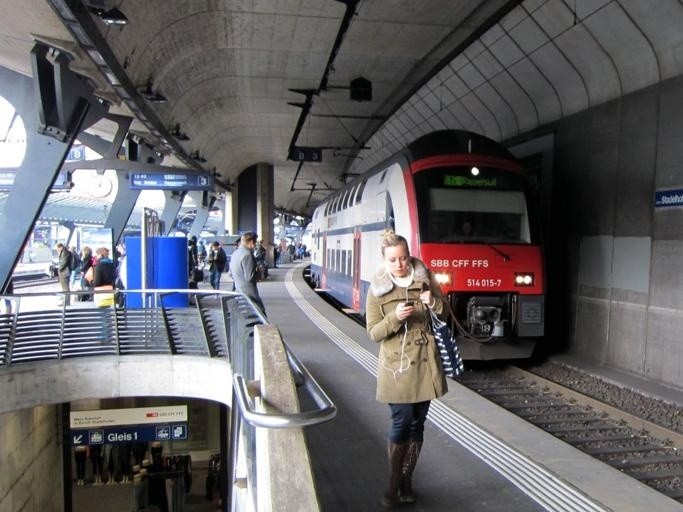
(70, 252), (79, 270)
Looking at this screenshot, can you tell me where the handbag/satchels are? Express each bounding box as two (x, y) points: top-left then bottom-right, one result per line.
(192, 270), (203, 282)
(93, 285), (114, 307)
(431, 320), (465, 378)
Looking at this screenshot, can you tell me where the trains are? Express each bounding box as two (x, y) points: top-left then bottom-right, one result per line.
(311, 129), (545, 360)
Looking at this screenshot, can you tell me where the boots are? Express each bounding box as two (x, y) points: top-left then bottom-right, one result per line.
(379, 440), (422, 507)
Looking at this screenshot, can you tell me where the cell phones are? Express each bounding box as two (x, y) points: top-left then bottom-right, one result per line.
(405, 301), (414, 307)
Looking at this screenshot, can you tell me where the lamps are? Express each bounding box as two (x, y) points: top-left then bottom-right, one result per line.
(140, 87), (166, 103)
(169, 123), (208, 166)
(89, 6), (129, 27)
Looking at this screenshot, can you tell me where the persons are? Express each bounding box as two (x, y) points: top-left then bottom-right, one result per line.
(74, 441), (164, 512)
(53, 235), (307, 307)
(230, 230), (267, 337)
(365, 228), (451, 508)
